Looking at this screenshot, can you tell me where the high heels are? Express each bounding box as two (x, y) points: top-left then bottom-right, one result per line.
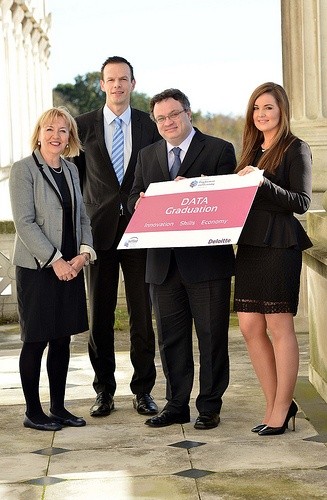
(251, 399), (299, 437)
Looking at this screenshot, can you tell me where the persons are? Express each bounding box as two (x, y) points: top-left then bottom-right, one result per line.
(127, 90), (239, 429)
(174, 80), (313, 435)
(9, 108), (96, 431)
(74, 56), (164, 417)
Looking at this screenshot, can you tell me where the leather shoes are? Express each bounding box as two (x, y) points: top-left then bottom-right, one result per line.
(132, 392), (159, 415)
(193, 412), (221, 430)
(143, 406), (191, 428)
(88, 391), (115, 418)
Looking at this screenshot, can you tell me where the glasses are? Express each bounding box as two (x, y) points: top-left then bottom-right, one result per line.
(154, 109), (186, 123)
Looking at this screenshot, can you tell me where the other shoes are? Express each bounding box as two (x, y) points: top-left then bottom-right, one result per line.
(46, 409), (87, 428)
(22, 411), (63, 432)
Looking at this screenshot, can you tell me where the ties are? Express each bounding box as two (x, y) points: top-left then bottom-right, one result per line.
(169, 147), (182, 180)
(110, 118), (125, 187)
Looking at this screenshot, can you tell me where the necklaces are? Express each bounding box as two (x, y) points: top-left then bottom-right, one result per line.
(46, 161), (63, 173)
(262, 144), (267, 152)
